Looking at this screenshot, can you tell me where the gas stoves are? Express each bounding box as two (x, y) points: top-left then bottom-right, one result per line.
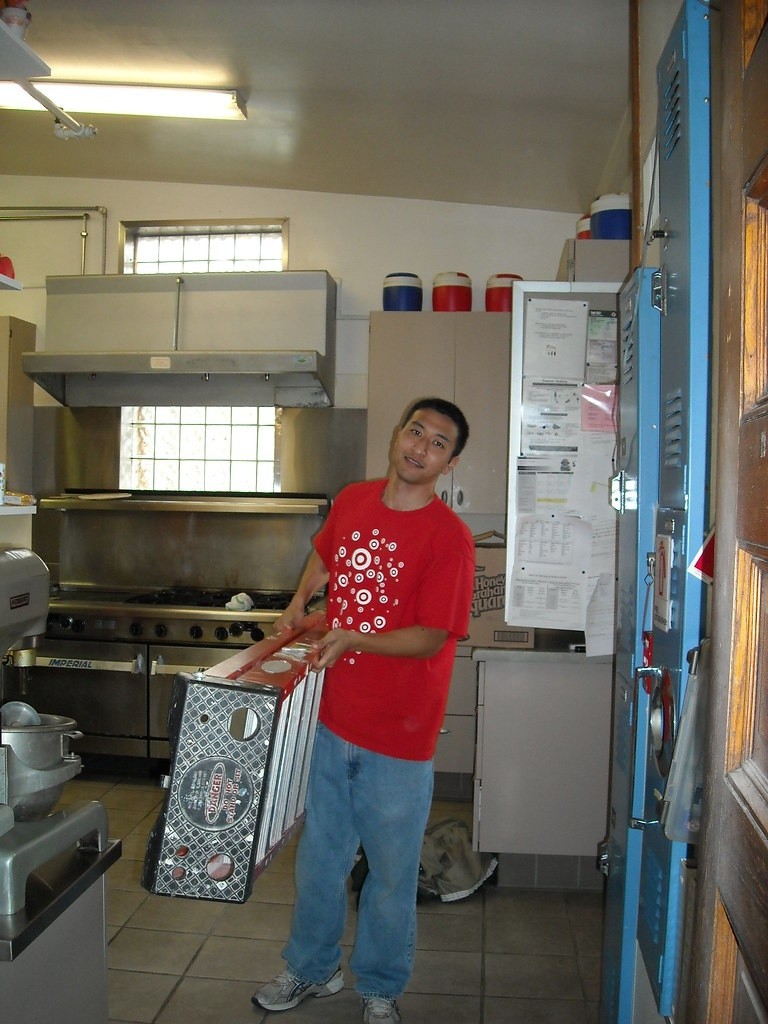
(130, 589), (318, 611)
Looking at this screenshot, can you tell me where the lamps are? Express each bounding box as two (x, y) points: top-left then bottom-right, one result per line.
(0, 79), (248, 122)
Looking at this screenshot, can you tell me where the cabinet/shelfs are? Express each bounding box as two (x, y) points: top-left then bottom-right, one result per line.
(553, 239), (630, 283)
(0, 315), (36, 499)
(364, 311), (511, 548)
(433, 648), (477, 774)
(471, 648), (610, 857)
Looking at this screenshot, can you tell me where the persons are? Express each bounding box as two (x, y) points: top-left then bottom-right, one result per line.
(250, 398), (474, 1023)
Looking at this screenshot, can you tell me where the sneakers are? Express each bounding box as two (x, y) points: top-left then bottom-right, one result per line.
(251, 963), (344, 1011)
(360, 997), (401, 1024)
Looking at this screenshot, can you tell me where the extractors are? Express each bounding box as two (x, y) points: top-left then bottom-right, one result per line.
(21, 274), (338, 408)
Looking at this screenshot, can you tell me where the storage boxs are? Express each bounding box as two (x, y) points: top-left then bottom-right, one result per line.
(456, 546), (536, 649)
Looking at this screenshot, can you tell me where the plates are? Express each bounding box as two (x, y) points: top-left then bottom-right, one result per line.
(78, 493), (132, 499)
